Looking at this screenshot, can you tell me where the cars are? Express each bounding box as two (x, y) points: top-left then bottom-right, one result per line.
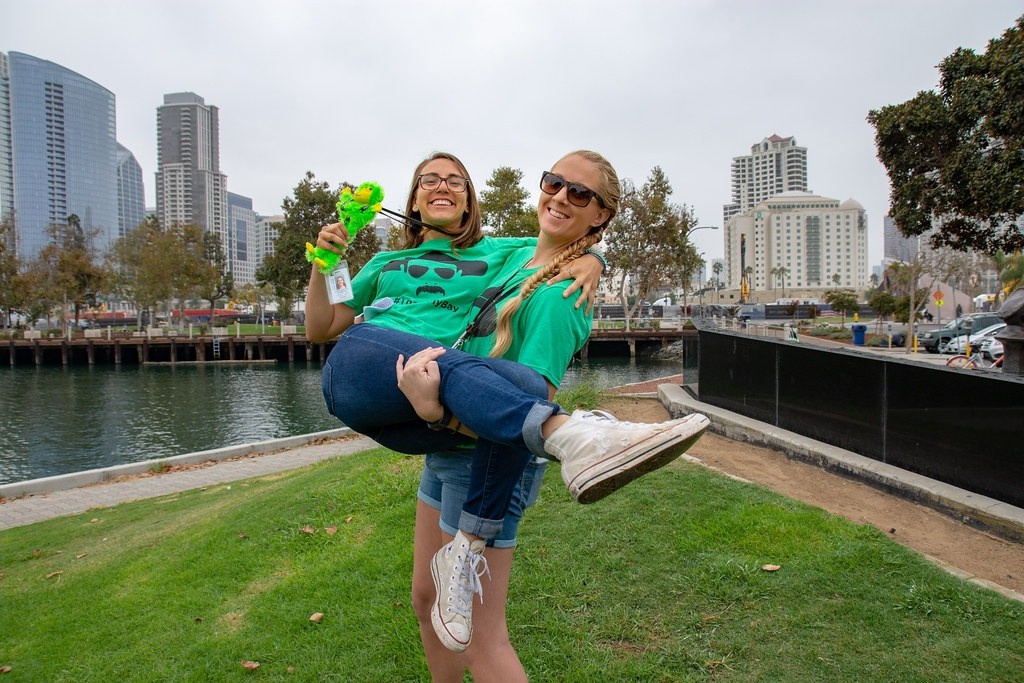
(25, 317), (101, 331)
(945, 322), (1008, 358)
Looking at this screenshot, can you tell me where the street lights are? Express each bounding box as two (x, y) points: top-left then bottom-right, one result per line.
(682, 225), (719, 316)
(697, 251), (707, 316)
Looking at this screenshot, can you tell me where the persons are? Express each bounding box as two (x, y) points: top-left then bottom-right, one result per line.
(337, 277), (346, 289)
(353, 150), (619, 683)
(305, 153), (710, 504)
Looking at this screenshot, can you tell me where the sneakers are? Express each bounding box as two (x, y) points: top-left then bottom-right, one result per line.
(428, 528), (491, 653)
(543, 401), (710, 504)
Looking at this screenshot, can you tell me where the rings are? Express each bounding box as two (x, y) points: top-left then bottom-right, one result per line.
(568, 269), (572, 278)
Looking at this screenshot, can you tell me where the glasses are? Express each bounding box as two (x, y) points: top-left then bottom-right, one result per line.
(417, 173), (469, 193)
(540, 171), (605, 210)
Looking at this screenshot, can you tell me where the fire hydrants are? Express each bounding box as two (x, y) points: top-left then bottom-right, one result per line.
(852, 312), (859, 322)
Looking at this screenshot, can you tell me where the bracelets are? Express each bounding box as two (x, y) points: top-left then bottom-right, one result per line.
(451, 420), (462, 435)
(589, 252), (605, 268)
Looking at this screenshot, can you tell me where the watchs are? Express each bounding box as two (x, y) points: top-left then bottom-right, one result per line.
(427, 405), (452, 431)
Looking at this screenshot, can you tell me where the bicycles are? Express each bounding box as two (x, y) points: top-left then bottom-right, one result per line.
(945, 343), (1005, 371)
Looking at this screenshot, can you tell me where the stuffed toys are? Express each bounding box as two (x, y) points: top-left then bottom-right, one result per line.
(306, 183), (383, 272)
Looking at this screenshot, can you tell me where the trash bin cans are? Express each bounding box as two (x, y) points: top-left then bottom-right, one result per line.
(852, 325), (867, 347)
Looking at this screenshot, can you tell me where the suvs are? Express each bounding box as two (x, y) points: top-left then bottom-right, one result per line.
(919, 310), (1006, 354)
